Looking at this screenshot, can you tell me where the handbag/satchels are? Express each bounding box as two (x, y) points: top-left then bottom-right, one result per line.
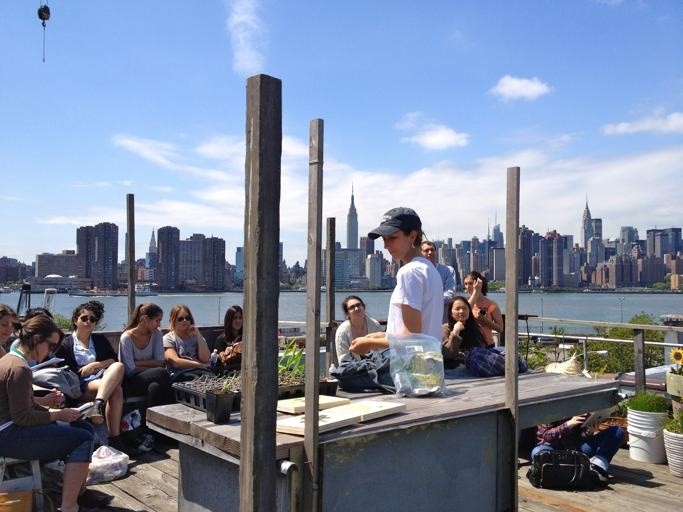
(330, 348), (396, 394)
(466, 346), (528, 378)
(0, 489), (54, 512)
(30, 357), (82, 399)
(526, 449), (600, 491)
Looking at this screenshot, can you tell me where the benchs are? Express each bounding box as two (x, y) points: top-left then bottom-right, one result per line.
(55, 326), (226, 412)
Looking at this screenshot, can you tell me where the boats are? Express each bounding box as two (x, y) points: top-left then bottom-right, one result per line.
(68, 282), (159, 296)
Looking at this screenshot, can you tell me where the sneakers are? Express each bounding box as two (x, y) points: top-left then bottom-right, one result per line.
(139, 435), (154, 452)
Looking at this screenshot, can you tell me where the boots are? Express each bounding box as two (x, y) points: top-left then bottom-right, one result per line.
(85, 400), (105, 425)
(108, 435), (140, 458)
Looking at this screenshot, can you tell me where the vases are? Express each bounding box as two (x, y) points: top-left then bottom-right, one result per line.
(666, 369), (683, 397)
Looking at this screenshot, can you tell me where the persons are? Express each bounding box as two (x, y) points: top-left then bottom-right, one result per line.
(212, 305), (243, 375)
(440, 296), (486, 370)
(530, 412), (621, 490)
(162, 304), (217, 381)
(463, 271), (504, 349)
(117, 303), (175, 454)
(420, 240), (457, 323)
(54, 300), (142, 459)
(334, 295), (383, 365)
(1, 306), (95, 511)
(347, 207), (444, 387)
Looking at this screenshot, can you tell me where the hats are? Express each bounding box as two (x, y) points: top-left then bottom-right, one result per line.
(368, 208), (421, 240)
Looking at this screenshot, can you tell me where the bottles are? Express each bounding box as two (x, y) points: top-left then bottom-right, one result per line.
(209, 348), (219, 367)
(480, 307), (486, 315)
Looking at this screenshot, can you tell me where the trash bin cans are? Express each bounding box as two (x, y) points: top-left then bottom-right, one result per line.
(664, 321), (683, 365)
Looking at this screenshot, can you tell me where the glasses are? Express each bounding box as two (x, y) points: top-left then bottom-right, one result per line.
(81, 315), (97, 322)
(178, 316), (190, 322)
(44, 338), (58, 352)
(347, 302), (361, 311)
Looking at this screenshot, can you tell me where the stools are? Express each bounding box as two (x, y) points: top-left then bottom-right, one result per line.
(0, 456), (48, 512)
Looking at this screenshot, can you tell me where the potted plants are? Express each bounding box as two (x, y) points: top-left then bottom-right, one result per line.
(597, 389), (683, 479)
(205, 372), (238, 423)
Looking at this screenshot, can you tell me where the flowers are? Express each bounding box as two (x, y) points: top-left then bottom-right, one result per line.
(668, 347), (683, 375)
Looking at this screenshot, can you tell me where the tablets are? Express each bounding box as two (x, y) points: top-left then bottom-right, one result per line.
(581, 406), (617, 429)
